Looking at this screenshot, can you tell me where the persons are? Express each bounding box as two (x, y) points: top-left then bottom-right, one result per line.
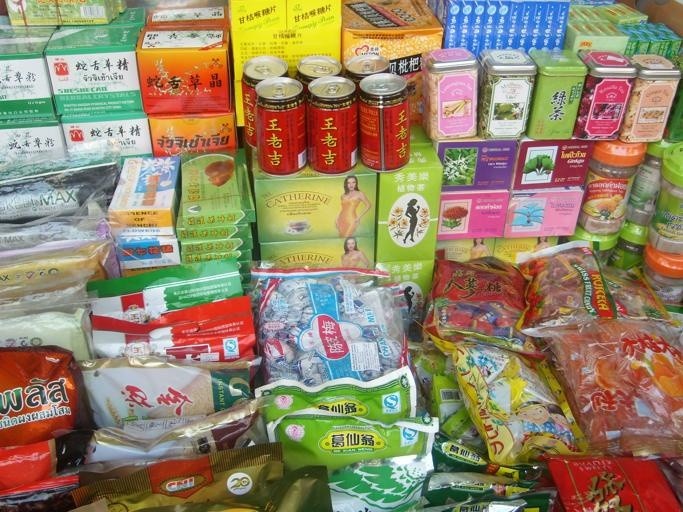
(335, 176), (371, 239)
(341, 237), (372, 270)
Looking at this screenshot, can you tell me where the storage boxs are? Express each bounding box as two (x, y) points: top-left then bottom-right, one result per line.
(0, 0), (593, 344)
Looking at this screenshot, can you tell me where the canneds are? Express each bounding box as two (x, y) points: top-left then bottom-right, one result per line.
(293, 56), (343, 95)
(256, 78), (308, 175)
(357, 73), (411, 172)
(242, 55), (291, 151)
(304, 77), (359, 174)
(344, 55), (391, 95)
